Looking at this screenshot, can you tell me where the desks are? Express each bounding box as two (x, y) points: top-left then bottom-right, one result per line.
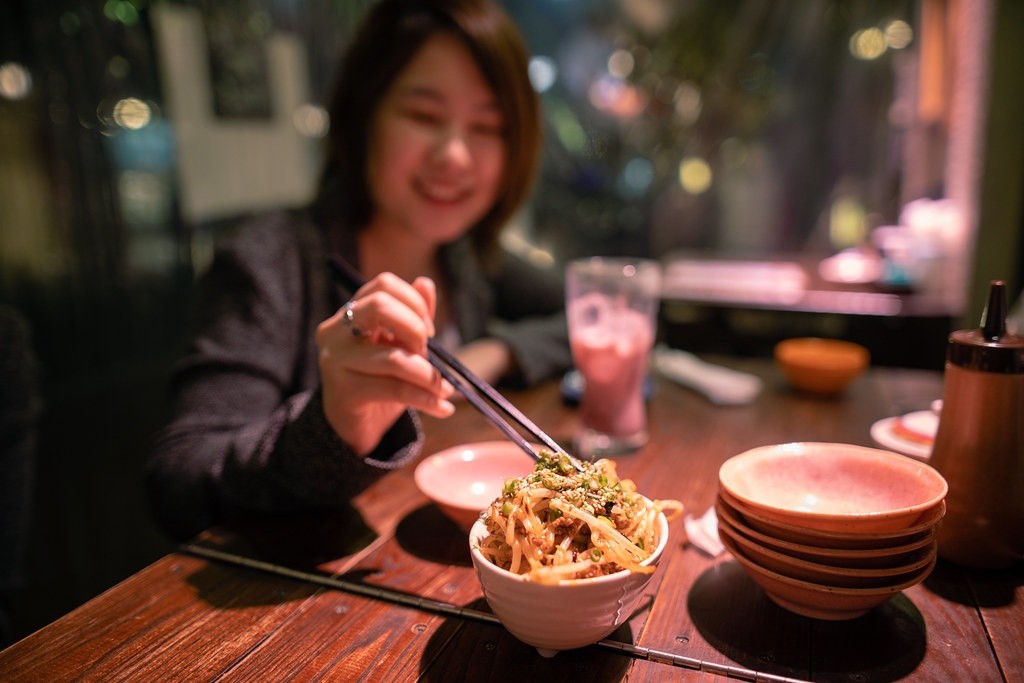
(0, 548), (746, 683)
(178, 353), (1024, 683)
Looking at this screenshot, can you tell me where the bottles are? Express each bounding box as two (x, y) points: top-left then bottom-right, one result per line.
(929, 280), (1024, 572)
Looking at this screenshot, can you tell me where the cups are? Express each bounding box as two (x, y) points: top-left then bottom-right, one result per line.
(565, 257), (660, 455)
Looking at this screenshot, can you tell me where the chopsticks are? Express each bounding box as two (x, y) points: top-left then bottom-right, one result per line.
(331, 253), (584, 480)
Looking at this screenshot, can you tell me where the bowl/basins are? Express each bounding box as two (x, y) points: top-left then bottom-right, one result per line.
(415, 440), (556, 533)
(715, 443), (948, 621)
(778, 339), (868, 396)
(468, 494), (668, 651)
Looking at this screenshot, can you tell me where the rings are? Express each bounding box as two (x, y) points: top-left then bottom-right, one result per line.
(343, 299), (374, 341)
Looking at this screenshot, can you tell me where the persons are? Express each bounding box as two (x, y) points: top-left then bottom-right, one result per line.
(143, 0), (576, 568)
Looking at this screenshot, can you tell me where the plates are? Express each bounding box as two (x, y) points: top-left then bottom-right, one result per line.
(870, 410), (940, 458)
